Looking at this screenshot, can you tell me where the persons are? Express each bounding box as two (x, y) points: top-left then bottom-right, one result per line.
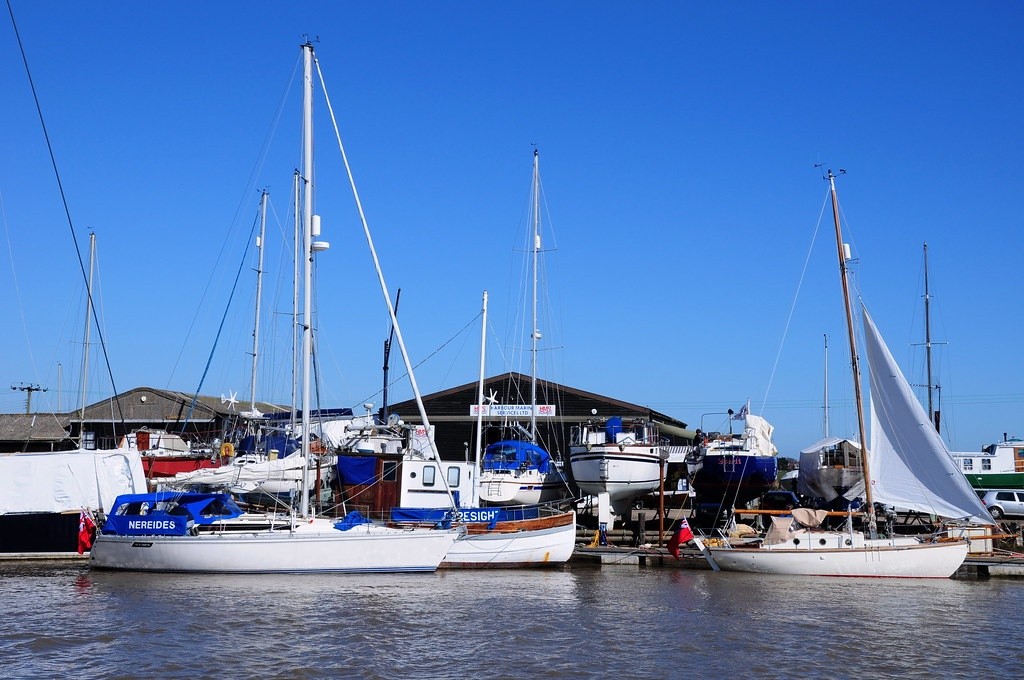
(693, 428), (706, 446)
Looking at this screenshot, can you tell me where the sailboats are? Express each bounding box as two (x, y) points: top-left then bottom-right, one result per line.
(710, 164), (998, 580)
(0, 35), (580, 571)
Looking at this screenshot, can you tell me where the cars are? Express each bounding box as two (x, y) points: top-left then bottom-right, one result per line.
(759, 490), (800, 520)
(983, 490), (1024, 519)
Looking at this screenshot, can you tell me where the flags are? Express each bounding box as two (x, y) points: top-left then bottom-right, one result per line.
(666, 520), (694, 561)
(78, 509), (95, 556)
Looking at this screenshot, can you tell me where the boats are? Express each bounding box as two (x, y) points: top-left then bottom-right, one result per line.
(947, 433), (1024, 497)
(798, 332), (871, 531)
(478, 440), (566, 507)
(686, 415), (778, 512)
(569, 417), (670, 518)
